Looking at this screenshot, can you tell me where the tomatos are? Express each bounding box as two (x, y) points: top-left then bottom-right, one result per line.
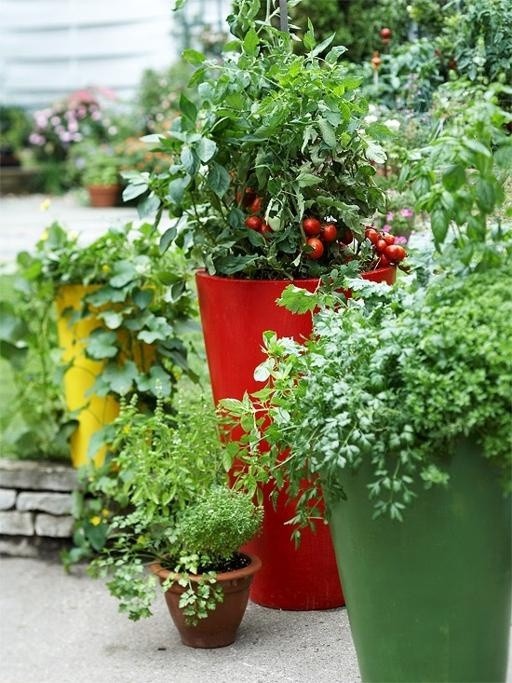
(236, 187), (406, 267)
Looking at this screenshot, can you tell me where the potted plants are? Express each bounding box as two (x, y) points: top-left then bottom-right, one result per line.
(120, 0), (413, 612)
(81, 155), (121, 207)
(87, 378), (264, 648)
(216, 260), (511, 683)
(1, 220), (208, 472)
